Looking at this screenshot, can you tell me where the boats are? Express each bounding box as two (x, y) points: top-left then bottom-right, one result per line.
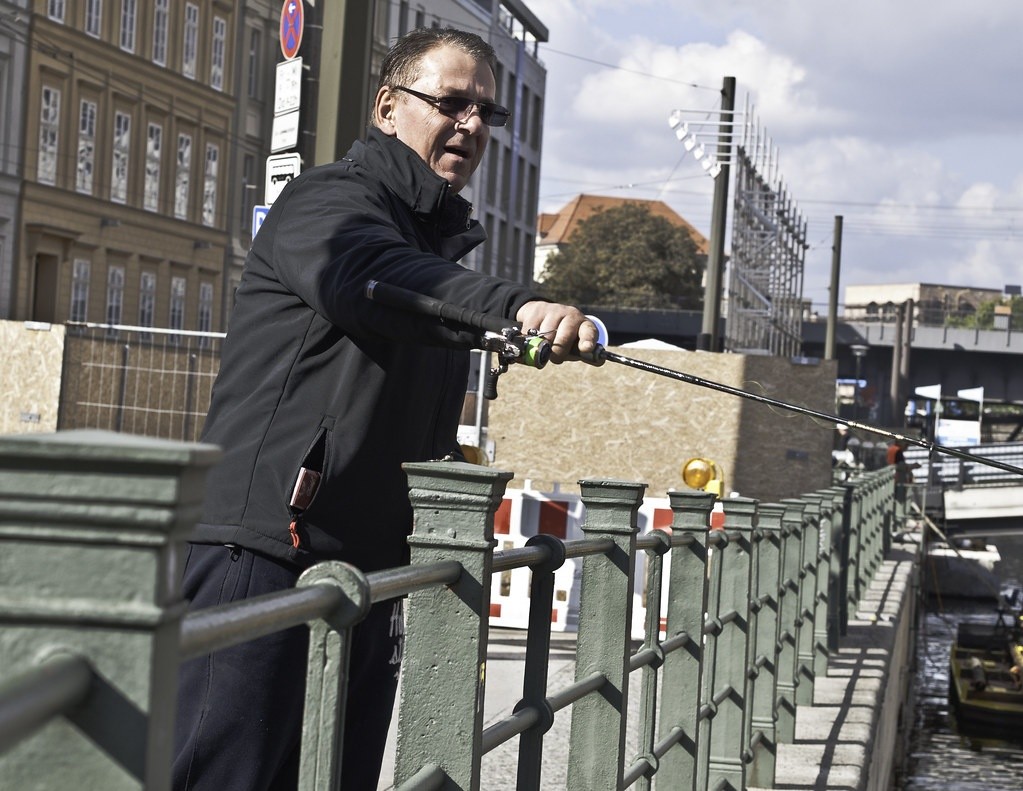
(943, 587), (1023, 744)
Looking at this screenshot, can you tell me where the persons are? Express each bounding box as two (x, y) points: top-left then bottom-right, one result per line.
(168, 23), (600, 791)
(886, 437), (923, 542)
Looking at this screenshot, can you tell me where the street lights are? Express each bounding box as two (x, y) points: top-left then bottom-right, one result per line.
(850, 345), (869, 419)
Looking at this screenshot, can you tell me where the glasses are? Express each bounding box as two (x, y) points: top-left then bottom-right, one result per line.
(391, 85), (511, 128)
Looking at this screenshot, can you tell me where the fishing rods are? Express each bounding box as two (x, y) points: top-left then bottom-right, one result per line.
(362, 274), (1023, 479)
(911, 500), (1019, 619)
